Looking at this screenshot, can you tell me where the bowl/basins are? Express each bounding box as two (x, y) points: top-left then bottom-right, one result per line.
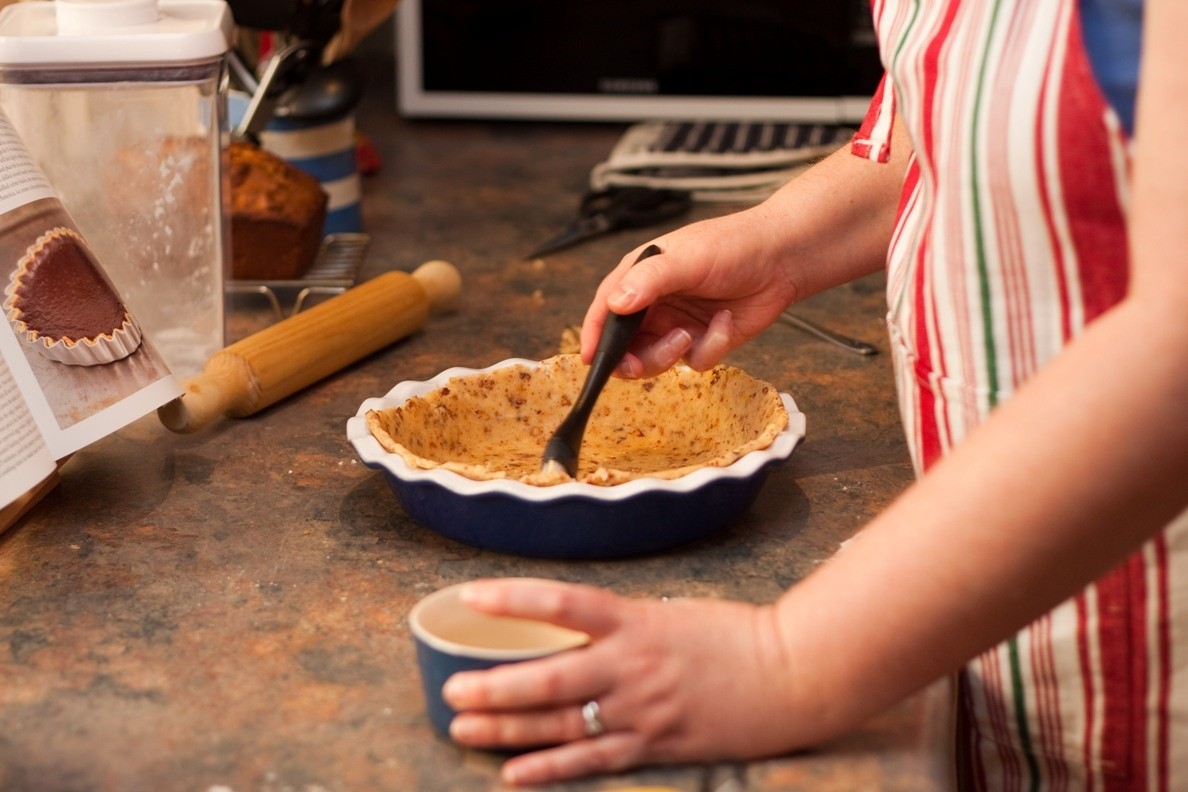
(344, 353), (809, 562)
(405, 576), (591, 738)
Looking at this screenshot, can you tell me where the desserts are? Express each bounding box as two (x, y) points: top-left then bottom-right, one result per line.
(5, 227), (142, 366)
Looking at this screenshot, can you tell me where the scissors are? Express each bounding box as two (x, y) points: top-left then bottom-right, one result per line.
(526, 185), (692, 262)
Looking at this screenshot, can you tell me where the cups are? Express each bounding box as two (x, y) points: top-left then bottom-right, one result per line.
(228, 76), (360, 237)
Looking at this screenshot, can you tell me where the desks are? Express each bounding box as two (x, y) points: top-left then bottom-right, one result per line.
(1, 56), (963, 792)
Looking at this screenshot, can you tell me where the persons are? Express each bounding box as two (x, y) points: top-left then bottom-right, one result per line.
(438, 1), (1188, 792)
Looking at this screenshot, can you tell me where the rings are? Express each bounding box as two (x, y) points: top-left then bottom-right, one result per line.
(582, 702), (603, 738)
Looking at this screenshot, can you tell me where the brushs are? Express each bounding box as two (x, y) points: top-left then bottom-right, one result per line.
(541, 243), (661, 478)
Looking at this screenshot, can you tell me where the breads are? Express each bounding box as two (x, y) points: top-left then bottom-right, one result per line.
(220, 140), (330, 278)
